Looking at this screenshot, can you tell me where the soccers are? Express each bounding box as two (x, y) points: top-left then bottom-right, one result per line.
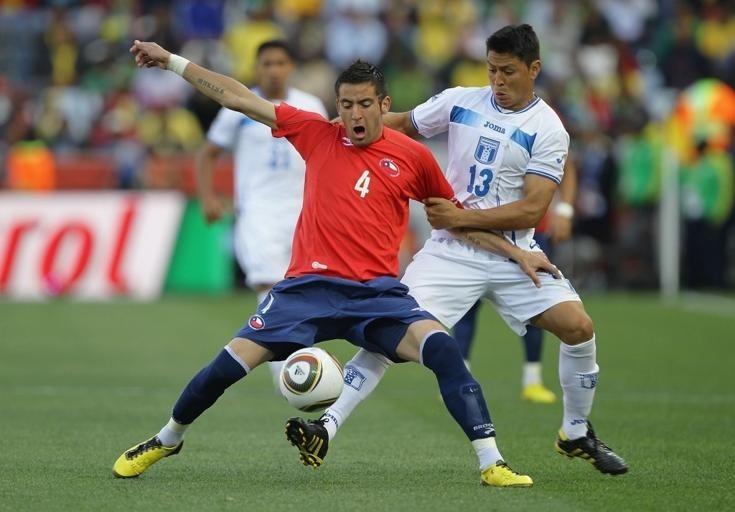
(280, 348), (343, 413)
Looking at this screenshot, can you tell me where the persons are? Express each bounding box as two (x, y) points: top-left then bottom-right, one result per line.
(226, 1), (518, 116)
(113, 37), (559, 487)
(282, 17), (628, 477)
(1, 1), (236, 188)
(194, 42), (329, 396)
(447, 86), (576, 405)
(519, 1), (733, 296)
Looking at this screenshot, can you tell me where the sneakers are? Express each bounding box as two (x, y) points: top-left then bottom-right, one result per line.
(113, 433), (183, 478)
(480, 460), (534, 487)
(285, 417), (328, 468)
(523, 383), (555, 403)
(556, 427), (628, 474)
